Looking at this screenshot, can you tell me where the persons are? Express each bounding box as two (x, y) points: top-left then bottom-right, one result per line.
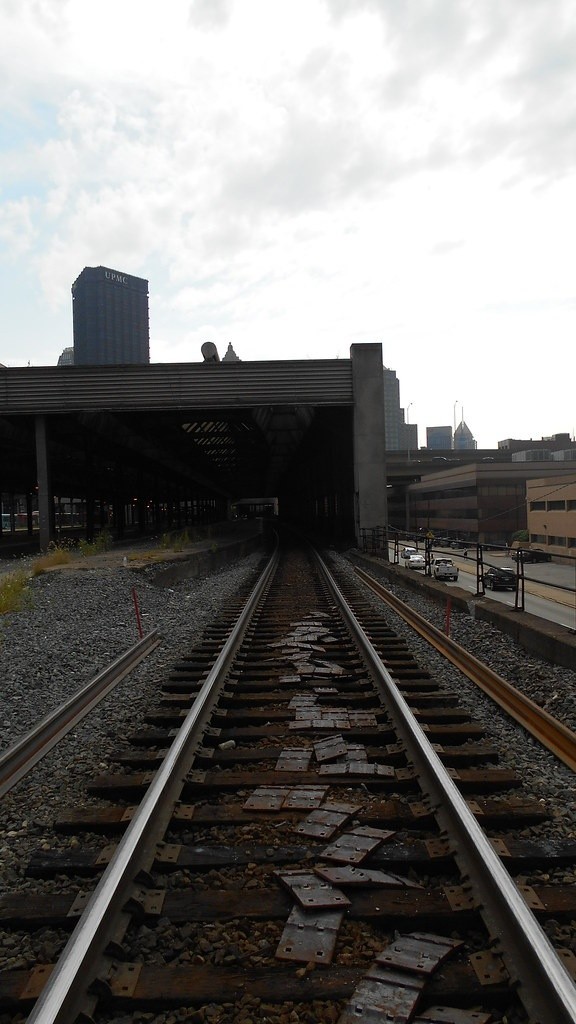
(463, 548), (468, 560)
(425, 532), (435, 543)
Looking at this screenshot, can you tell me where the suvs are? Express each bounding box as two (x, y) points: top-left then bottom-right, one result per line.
(481, 566), (517, 592)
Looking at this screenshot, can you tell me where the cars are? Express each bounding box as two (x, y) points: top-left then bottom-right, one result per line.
(404, 554), (426, 570)
(400, 546), (417, 559)
(513, 548), (551, 563)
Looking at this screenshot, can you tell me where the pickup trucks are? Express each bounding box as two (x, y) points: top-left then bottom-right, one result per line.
(425, 557), (459, 581)
